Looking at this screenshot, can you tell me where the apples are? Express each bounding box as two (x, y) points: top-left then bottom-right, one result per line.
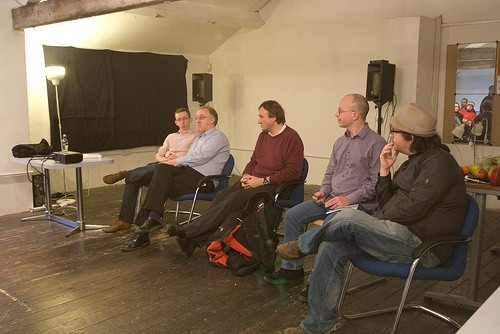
(471, 163), (500, 186)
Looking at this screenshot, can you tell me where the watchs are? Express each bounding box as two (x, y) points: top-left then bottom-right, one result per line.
(263, 177), (269, 187)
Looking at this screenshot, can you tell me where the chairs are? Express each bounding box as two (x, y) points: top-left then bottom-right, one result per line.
(137, 154), (480, 334)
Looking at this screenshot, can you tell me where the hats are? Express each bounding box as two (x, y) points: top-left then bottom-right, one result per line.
(467, 101), (475, 107)
(391, 103), (438, 137)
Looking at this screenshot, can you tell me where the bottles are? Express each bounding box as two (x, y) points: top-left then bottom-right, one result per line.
(62, 134), (68, 152)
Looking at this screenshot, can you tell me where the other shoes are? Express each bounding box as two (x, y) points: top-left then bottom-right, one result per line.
(264, 268), (304, 285)
(103, 172), (123, 184)
(276, 240), (305, 259)
(169, 235), (196, 258)
(102, 221), (129, 232)
(284, 326), (305, 334)
(167, 224), (185, 239)
(299, 285), (311, 303)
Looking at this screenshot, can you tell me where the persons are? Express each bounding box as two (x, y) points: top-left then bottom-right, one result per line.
(102, 106), (197, 234)
(453, 86), (494, 145)
(264, 92), (390, 302)
(283, 101), (470, 334)
(168, 101), (304, 255)
(122, 106), (230, 252)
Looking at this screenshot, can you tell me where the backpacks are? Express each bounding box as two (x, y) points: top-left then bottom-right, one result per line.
(206, 191), (284, 275)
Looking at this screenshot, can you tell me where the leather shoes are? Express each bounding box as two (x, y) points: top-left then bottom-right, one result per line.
(135, 216), (163, 233)
(120, 233), (150, 250)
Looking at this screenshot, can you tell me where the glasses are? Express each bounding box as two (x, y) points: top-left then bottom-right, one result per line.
(390, 126), (406, 135)
(195, 115), (215, 120)
(338, 106), (356, 114)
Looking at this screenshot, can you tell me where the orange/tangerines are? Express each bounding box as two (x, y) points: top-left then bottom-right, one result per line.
(462, 165), (469, 174)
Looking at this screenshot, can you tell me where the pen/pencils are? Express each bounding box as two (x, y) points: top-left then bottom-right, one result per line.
(313, 193), (323, 203)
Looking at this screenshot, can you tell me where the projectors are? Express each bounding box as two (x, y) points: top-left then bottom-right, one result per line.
(50, 152), (83, 165)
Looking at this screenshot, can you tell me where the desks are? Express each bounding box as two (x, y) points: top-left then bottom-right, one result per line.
(21, 158), (114, 237)
(424, 180), (500, 334)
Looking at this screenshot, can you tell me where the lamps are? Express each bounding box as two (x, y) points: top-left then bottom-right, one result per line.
(451, 119), (476, 165)
(45, 66), (76, 205)
(471, 117), (488, 144)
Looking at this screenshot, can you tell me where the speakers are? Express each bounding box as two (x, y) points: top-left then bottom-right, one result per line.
(365, 60), (396, 105)
(192, 73), (212, 102)
